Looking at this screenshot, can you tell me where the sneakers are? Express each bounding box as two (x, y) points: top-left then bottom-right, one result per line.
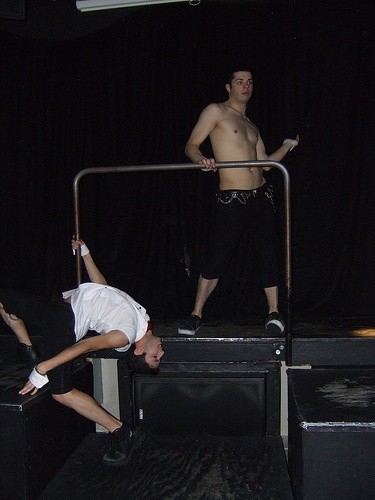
(103, 421), (146, 462)
(264, 312), (285, 332)
(177, 315), (202, 335)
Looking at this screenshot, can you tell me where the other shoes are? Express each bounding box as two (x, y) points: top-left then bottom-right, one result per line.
(15, 343), (40, 361)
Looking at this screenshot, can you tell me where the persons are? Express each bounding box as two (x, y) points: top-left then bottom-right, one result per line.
(178, 62), (300, 335)
(0, 237), (164, 467)
